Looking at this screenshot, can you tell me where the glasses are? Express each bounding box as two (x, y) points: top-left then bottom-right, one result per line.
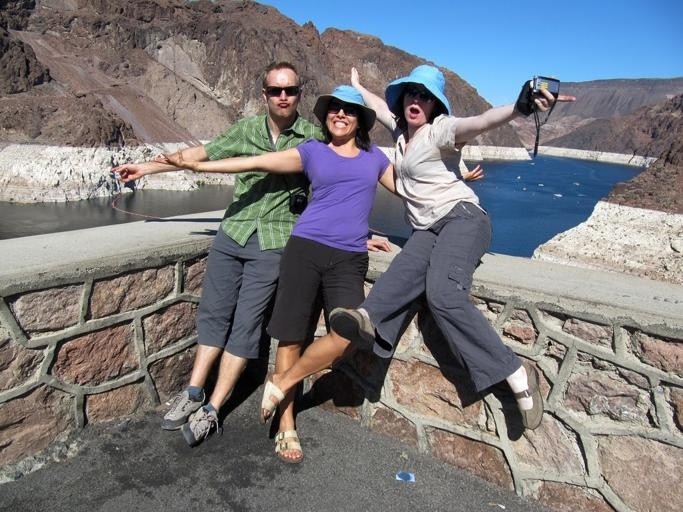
(406, 86), (432, 103)
(266, 84), (297, 98)
(327, 101), (359, 116)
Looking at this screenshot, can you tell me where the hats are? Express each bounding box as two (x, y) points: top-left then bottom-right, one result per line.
(383, 64), (452, 117)
(311, 84), (376, 133)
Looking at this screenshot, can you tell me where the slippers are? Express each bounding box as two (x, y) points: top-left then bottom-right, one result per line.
(258, 371), (287, 425)
(274, 424), (305, 466)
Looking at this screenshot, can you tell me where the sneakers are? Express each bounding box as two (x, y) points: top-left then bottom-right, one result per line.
(327, 305), (376, 358)
(513, 360), (544, 429)
(159, 388), (208, 432)
(182, 404), (220, 447)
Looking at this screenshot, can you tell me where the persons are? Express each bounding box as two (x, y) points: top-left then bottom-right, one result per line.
(110, 60), (325, 448)
(259, 64), (577, 430)
(153, 83), (485, 465)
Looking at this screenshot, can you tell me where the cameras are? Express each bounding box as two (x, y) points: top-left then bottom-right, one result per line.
(289, 195), (308, 214)
(533, 75), (560, 99)
